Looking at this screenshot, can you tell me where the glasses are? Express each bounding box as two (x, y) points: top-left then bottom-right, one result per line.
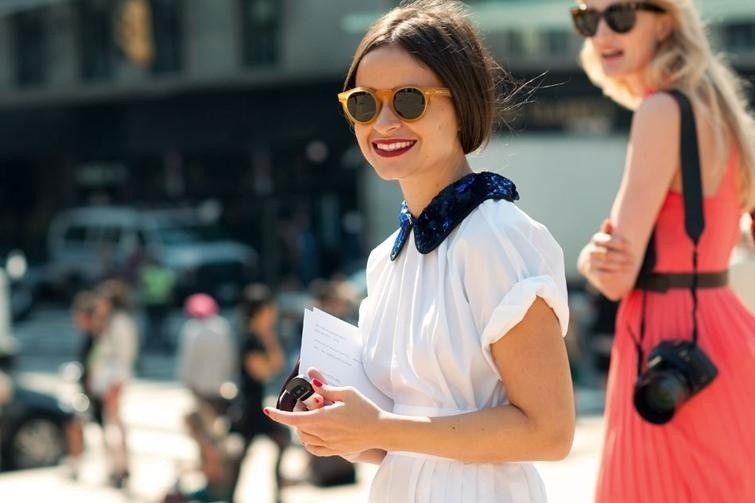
(572, 4), (665, 37)
(338, 85), (451, 123)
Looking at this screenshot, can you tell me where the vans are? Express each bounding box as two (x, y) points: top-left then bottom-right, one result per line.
(43, 205), (261, 311)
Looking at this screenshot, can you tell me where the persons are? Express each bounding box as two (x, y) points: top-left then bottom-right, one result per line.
(567, 0), (755, 503)
(263, 5), (577, 503)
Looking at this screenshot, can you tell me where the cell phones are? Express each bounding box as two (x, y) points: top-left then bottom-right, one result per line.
(286, 374), (335, 411)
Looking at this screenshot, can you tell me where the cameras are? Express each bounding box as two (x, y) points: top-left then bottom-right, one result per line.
(633, 339), (717, 424)
